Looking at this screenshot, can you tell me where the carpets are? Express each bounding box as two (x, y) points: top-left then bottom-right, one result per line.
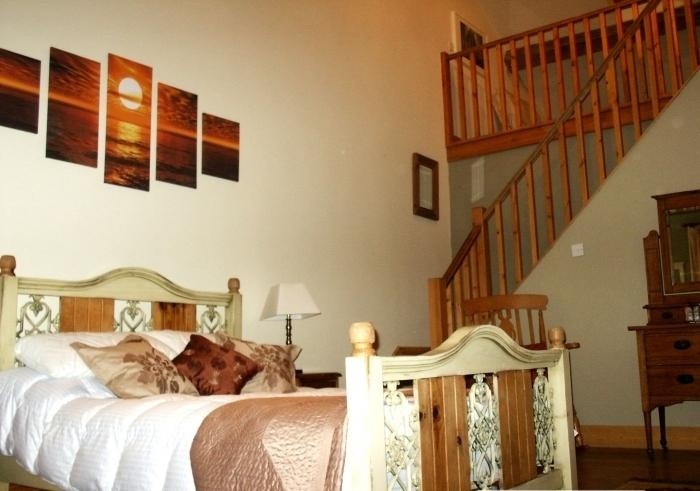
(616, 478), (700, 490)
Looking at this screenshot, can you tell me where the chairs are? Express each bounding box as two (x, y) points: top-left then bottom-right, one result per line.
(460, 295), (584, 449)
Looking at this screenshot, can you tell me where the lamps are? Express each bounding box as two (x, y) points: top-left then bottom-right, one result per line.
(261, 281), (322, 372)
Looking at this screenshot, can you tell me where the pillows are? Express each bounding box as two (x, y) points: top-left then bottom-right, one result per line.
(15, 329), (301, 399)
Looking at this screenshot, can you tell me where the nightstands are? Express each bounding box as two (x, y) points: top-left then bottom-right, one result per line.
(297, 373), (342, 388)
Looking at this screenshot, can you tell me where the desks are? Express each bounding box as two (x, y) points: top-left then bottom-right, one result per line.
(627, 304), (699, 455)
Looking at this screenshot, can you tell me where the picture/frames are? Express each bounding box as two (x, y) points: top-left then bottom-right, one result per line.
(452, 10), (489, 77)
(412, 153), (440, 221)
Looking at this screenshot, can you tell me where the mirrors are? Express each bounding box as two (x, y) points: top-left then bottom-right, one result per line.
(651, 190), (699, 295)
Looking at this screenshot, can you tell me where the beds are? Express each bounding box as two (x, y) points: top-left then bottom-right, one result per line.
(0, 255), (580, 491)
